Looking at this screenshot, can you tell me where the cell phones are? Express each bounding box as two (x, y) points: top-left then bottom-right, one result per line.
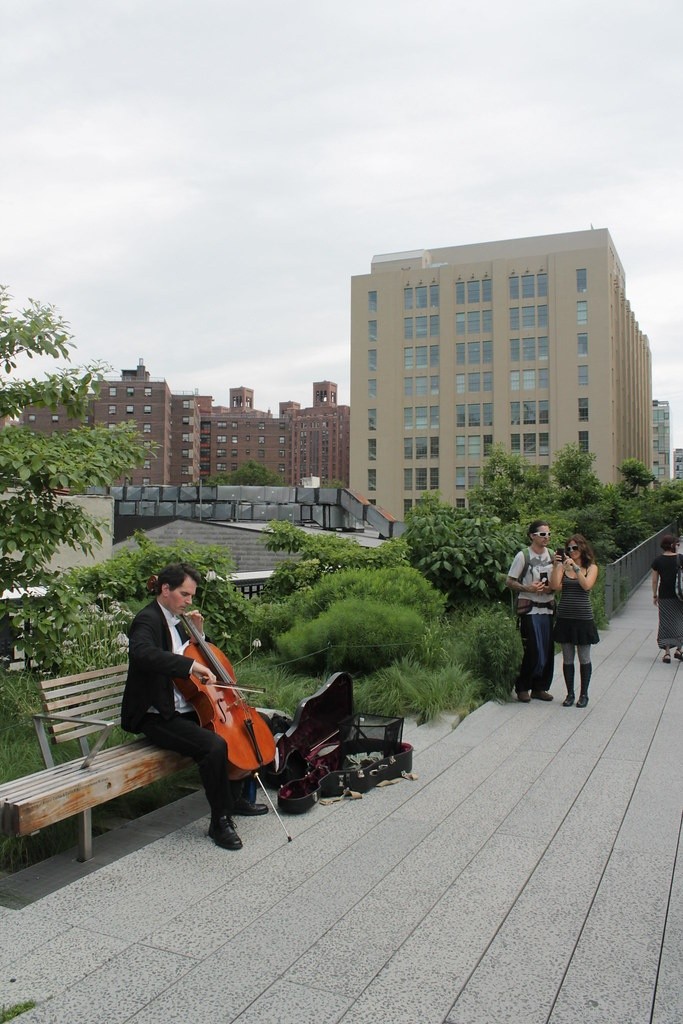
(540, 572), (548, 586)
(557, 547), (565, 561)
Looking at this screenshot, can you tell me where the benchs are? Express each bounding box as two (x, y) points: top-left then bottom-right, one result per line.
(0, 662), (195, 863)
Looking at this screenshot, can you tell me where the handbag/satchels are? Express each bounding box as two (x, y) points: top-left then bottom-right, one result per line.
(675, 570), (683, 601)
(516, 599), (533, 615)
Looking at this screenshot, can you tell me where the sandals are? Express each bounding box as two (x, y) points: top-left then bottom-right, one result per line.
(663, 654), (670, 663)
(674, 649), (683, 661)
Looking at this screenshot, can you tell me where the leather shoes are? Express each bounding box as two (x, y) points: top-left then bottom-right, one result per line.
(209, 815), (243, 850)
(231, 797), (268, 815)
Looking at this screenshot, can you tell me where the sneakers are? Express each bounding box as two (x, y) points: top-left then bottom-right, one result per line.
(531, 690), (553, 701)
(517, 691), (530, 702)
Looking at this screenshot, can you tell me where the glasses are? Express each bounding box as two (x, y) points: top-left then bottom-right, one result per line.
(567, 545), (578, 552)
(676, 543), (680, 546)
(533, 532), (551, 538)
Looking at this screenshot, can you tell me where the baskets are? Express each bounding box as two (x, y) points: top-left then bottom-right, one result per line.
(338, 713), (404, 758)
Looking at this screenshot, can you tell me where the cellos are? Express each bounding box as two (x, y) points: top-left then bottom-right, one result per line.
(146, 575), (292, 842)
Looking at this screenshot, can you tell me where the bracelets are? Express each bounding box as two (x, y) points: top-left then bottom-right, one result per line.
(574, 567), (581, 573)
(653, 595), (658, 598)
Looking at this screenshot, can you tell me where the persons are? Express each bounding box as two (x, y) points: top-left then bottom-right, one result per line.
(551, 533), (599, 709)
(650, 534), (683, 663)
(120, 561), (269, 851)
(505, 519), (563, 702)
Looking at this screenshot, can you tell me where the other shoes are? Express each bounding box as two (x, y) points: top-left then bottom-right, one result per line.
(563, 694), (575, 706)
(576, 695), (588, 707)
